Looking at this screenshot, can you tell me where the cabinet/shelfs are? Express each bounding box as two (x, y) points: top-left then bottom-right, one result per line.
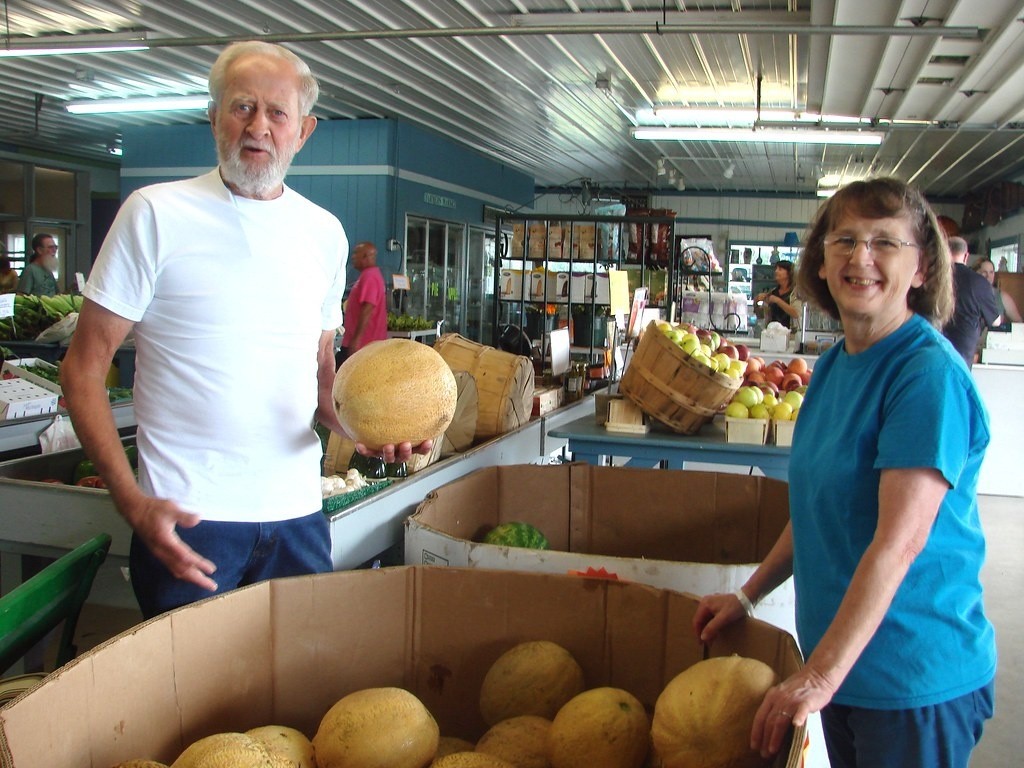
(491, 215), (678, 375)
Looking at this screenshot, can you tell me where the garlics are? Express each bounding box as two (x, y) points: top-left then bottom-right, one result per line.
(322, 469), (364, 496)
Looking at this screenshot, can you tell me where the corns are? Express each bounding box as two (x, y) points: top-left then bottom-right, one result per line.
(0, 294), (85, 340)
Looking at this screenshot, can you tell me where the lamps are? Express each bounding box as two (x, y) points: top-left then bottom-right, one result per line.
(816, 189), (837, 197)
(667, 168), (678, 185)
(630, 121), (888, 141)
(676, 176), (686, 191)
(723, 157), (736, 179)
(657, 159), (666, 175)
(1, 32), (150, 60)
(63, 95), (215, 116)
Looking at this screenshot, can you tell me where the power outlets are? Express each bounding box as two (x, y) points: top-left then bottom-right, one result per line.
(386, 239), (399, 252)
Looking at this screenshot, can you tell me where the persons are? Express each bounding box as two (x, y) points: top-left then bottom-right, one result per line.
(690, 179), (998, 768)
(0, 254), (18, 295)
(753, 260), (802, 354)
(60, 38), (434, 623)
(339, 243), (387, 373)
(19, 234), (62, 298)
(942, 237), (1023, 372)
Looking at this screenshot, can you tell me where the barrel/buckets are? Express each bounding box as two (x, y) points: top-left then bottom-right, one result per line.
(118, 347), (137, 389)
(617, 321), (744, 435)
(573, 315), (608, 348)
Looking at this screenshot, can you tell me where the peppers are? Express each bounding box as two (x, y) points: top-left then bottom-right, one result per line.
(15, 362), (133, 401)
(40, 445), (139, 489)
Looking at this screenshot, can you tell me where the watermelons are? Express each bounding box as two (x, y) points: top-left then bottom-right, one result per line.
(484, 521), (549, 551)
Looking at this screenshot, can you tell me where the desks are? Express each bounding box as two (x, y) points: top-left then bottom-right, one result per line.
(547, 413), (793, 485)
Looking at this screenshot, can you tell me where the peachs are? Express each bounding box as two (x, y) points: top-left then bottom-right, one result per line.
(743, 356), (813, 392)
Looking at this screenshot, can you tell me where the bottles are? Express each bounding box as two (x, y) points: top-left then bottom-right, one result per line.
(566, 362), (584, 402)
(348, 450), (407, 490)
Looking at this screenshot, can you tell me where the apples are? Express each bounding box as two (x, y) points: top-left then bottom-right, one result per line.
(725, 385), (808, 421)
(657, 322), (749, 378)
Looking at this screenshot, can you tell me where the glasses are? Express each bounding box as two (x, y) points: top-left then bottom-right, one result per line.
(42, 245), (58, 250)
(823, 236), (921, 255)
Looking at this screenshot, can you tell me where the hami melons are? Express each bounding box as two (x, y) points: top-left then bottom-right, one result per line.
(114, 640), (783, 768)
(332, 339), (457, 451)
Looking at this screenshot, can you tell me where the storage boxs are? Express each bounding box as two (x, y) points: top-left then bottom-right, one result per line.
(0, 219), (836, 767)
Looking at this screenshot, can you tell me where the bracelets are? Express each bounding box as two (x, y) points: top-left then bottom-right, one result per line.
(735, 588), (755, 617)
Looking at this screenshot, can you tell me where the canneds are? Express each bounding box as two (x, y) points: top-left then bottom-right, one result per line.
(542, 362), (610, 402)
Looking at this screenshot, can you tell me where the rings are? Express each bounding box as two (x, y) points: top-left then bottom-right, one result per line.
(779, 710), (793, 719)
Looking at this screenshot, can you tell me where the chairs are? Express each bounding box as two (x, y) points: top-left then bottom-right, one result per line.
(1, 533), (111, 670)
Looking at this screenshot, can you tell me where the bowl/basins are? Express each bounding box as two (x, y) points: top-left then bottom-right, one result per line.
(499, 324), (532, 359)
